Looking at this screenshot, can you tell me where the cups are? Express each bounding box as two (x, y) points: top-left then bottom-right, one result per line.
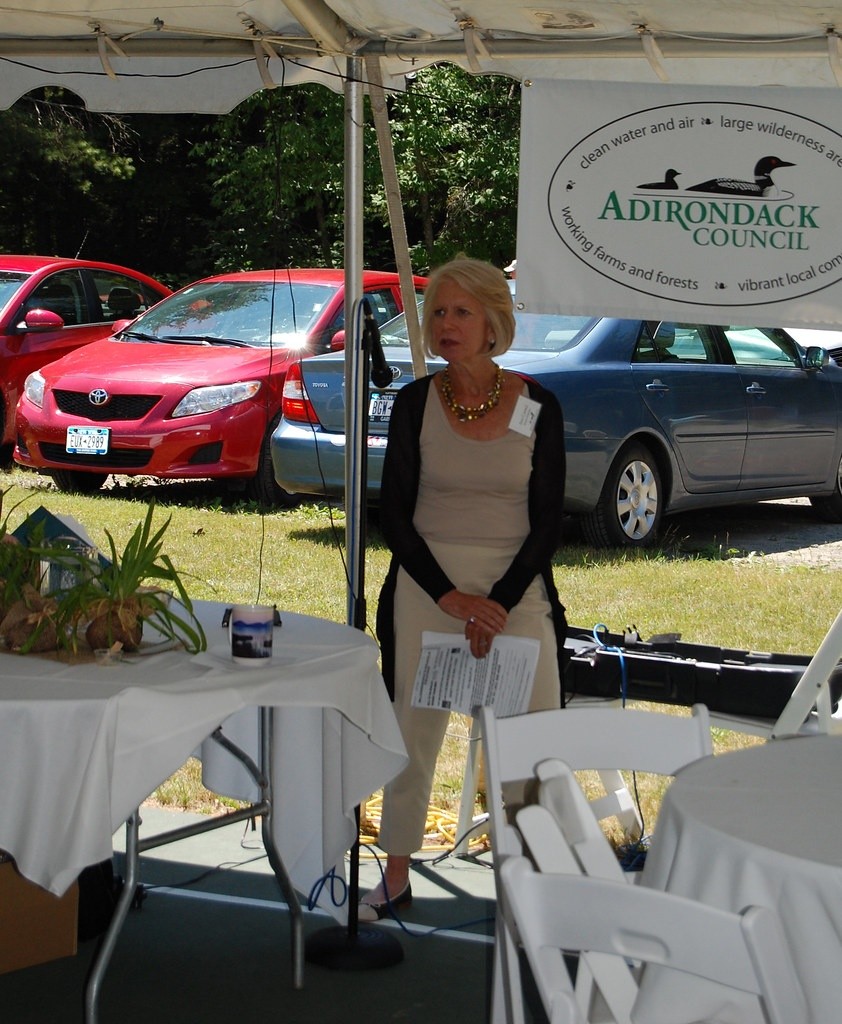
(228, 603), (275, 667)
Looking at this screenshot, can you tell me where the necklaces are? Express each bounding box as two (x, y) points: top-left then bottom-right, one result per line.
(440, 363), (503, 421)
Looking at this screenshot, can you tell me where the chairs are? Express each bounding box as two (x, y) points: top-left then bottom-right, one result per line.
(650, 321), (676, 361)
(467, 699), (813, 1024)
(43, 286), (80, 326)
(106, 287), (140, 318)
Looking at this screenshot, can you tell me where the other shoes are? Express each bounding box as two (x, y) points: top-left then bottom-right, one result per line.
(356, 881), (412, 919)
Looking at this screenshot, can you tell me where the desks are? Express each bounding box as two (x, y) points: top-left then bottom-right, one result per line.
(0, 600), (412, 1024)
(619, 735), (842, 1024)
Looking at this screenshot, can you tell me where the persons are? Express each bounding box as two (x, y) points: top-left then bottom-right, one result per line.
(357, 252), (569, 922)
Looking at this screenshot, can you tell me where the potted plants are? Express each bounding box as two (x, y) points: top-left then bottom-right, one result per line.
(11, 491), (206, 663)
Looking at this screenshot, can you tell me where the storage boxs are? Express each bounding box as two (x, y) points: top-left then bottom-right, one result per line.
(0, 847), (79, 977)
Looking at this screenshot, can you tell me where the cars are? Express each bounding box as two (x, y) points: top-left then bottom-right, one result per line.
(269, 278), (842, 552)
(0, 255), (215, 472)
(12, 263), (429, 509)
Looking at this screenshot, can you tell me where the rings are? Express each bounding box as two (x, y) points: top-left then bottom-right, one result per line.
(479, 641), (487, 648)
(470, 616), (476, 622)
